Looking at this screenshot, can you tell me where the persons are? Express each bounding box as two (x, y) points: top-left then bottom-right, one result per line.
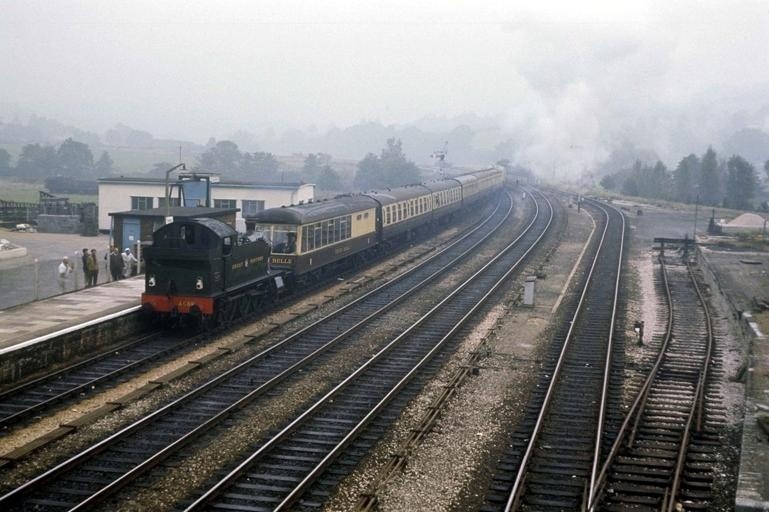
(81, 245), (138, 288)
(56, 256), (75, 292)
